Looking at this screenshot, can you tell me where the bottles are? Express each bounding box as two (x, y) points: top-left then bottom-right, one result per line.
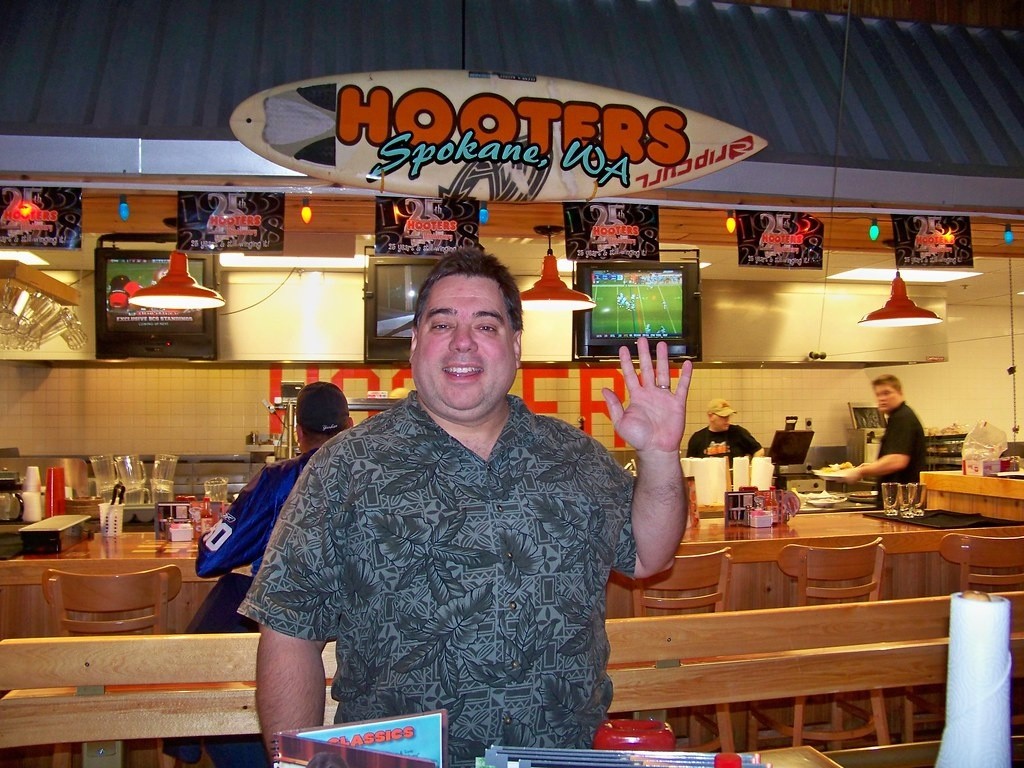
(200, 497), (214, 537)
(713, 752), (743, 768)
(765, 486), (779, 527)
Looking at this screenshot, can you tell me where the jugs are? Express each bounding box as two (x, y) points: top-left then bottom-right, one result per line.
(0, 468), (24, 522)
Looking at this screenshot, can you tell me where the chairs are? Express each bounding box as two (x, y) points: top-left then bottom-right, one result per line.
(900, 528), (1024, 744)
(627, 541), (744, 754)
(40, 567), (183, 768)
(745, 535), (893, 748)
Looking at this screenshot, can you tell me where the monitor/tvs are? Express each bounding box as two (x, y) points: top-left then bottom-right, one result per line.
(767, 429), (815, 466)
(94, 246), (220, 361)
(363, 256), (449, 364)
(575, 262), (699, 357)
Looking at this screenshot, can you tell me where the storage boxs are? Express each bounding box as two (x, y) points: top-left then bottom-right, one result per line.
(17, 510), (93, 557)
(170, 521), (193, 543)
(961, 457), (1019, 477)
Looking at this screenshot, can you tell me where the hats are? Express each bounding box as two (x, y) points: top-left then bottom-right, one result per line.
(708, 400), (738, 417)
(296, 381), (350, 432)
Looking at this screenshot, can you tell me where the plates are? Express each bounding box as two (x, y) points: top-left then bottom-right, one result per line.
(847, 490), (879, 503)
(805, 492), (848, 508)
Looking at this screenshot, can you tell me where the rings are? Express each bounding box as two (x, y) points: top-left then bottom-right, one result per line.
(657, 384), (670, 390)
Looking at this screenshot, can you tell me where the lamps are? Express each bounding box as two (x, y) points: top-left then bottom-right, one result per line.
(125, 216), (226, 312)
(857, 235), (943, 328)
(518, 224), (598, 314)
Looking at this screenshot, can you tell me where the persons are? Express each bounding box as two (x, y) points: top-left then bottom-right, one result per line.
(617, 293), (637, 313)
(661, 300), (667, 309)
(839, 376), (930, 508)
(238, 244), (694, 768)
(644, 323), (667, 334)
(687, 396), (767, 492)
(620, 272), (680, 290)
(196, 380), (356, 579)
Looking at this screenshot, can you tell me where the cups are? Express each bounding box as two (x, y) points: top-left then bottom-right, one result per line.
(0, 284), (89, 352)
(21, 467), (42, 523)
(881, 482), (928, 519)
(87, 451), (180, 506)
(204, 476), (230, 503)
